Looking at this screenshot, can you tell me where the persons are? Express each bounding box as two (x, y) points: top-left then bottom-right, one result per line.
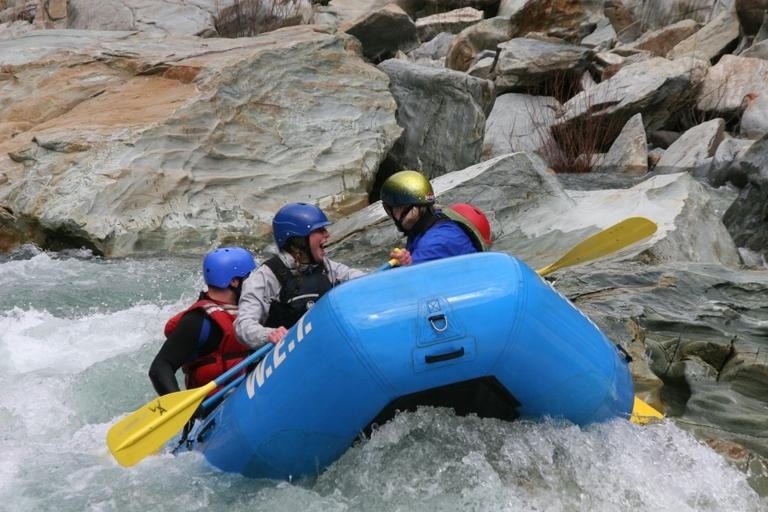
(381, 169), (491, 266)
(148, 247), (256, 422)
(232, 201), (412, 346)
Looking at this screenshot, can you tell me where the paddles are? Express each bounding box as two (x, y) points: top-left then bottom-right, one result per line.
(536, 216), (658, 276)
(106, 248), (405, 468)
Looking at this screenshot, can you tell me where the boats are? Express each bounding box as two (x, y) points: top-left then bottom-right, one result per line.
(155, 250), (635, 488)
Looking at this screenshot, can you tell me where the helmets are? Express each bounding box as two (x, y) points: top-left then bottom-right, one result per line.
(273, 202), (331, 249)
(380, 171), (435, 206)
(203, 247), (257, 289)
